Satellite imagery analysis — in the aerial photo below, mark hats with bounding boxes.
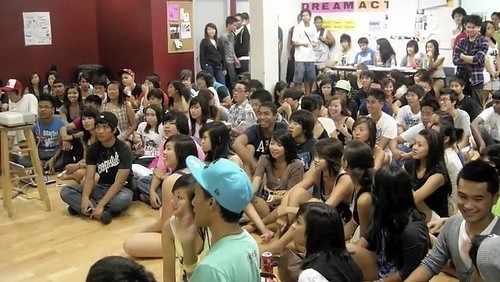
[186,155,254,213]
[476,233,500,282]
[333,80,351,92]
[119,69,134,76]
[96,111,120,136]
[1,79,22,92]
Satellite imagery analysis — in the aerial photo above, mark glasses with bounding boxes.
[232,89,247,93]
[38,105,53,109]
[405,94,416,99]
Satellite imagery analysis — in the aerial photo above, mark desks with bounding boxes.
[325,66,420,79]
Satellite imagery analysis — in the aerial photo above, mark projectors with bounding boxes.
[0,110,35,127]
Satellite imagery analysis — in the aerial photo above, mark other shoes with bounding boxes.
[140,193,150,203]
[69,206,77,214]
[101,210,112,225]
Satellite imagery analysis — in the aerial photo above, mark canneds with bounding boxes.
[261,252,273,274]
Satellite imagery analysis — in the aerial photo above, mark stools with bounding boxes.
[0,124,53,217]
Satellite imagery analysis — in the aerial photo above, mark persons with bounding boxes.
[0,63,500,282]
[373,38,397,67]
[401,41,424,67]
[422,39,446,99]
[452,7,500,107]
[354,37,378,65]
[336,33,356,65]
[285,9,335,95]
[199,13,249,86]
